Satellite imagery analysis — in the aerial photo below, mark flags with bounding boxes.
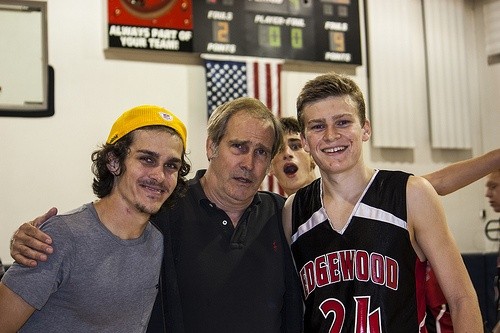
[205,59,285,198]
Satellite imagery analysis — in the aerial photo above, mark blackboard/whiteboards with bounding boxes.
[0,0,48,111]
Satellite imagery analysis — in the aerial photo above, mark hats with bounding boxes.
[106,104,187,152]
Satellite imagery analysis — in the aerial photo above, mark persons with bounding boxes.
[265,116,500,333]
[10,96,306,333]
[1,106,191,333]
[281,74,484,333]
